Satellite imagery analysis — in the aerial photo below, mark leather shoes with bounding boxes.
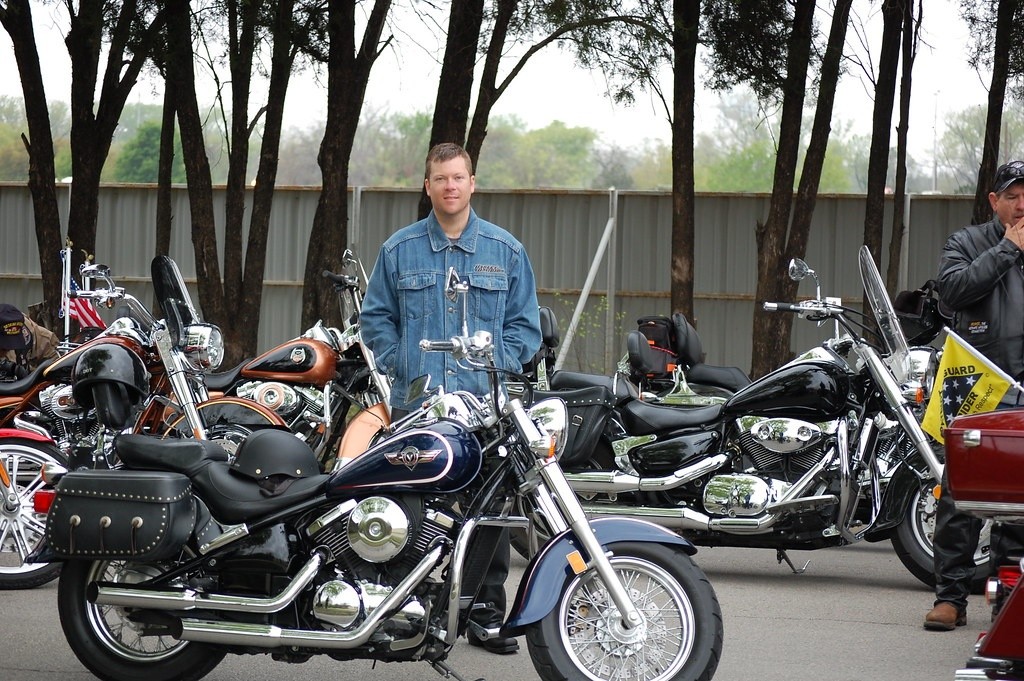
[466,622,520,653]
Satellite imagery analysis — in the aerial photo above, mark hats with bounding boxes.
[992,160,1024,193]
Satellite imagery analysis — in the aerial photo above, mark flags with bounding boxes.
[920,329,1012,446]
[58,272,106,329]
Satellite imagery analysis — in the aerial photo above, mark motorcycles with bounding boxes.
[0,235,1024,681]
[27,265,724,680]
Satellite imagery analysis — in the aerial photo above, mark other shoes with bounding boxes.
[923,601,967,629]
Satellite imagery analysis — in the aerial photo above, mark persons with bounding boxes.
[0,302,61,385]
[923,160,1024,630]
[357,141,543,653]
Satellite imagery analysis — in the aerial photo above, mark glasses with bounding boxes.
[992,165,1024,190]
